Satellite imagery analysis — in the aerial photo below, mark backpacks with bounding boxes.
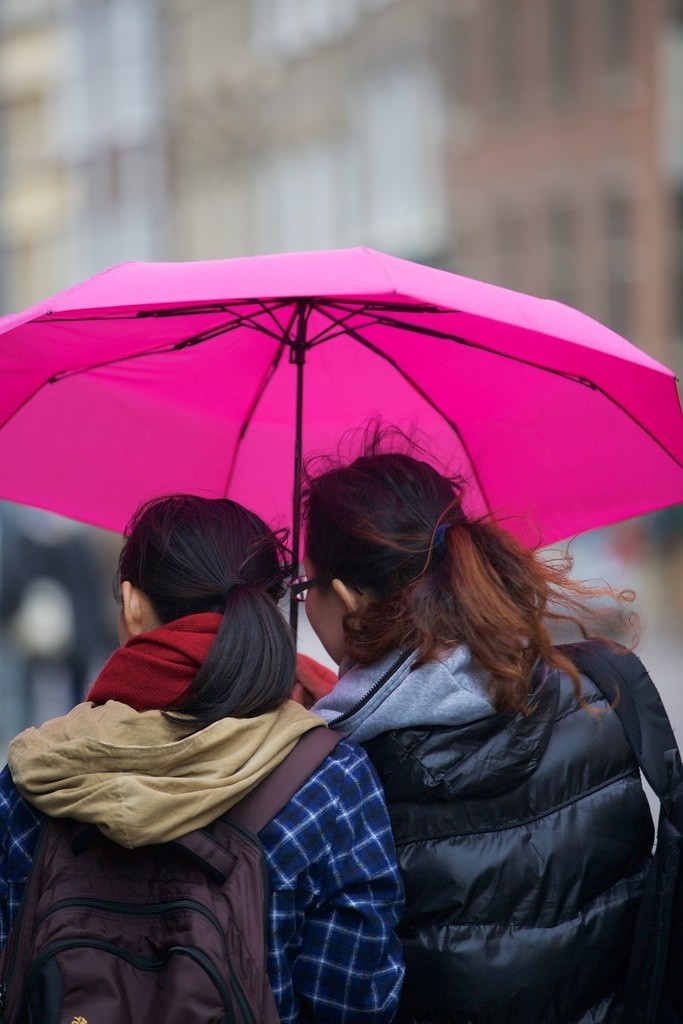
[5,727,343,1024]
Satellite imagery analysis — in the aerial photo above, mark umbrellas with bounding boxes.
[0,241,683,686]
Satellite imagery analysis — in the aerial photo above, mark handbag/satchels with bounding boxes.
[554,640,683,1024]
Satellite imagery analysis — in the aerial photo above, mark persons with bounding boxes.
[283,409,682,1023]
[0,492,407,1024]
[0,496,125,752]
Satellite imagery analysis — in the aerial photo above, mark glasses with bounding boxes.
[287,574,365,602]
